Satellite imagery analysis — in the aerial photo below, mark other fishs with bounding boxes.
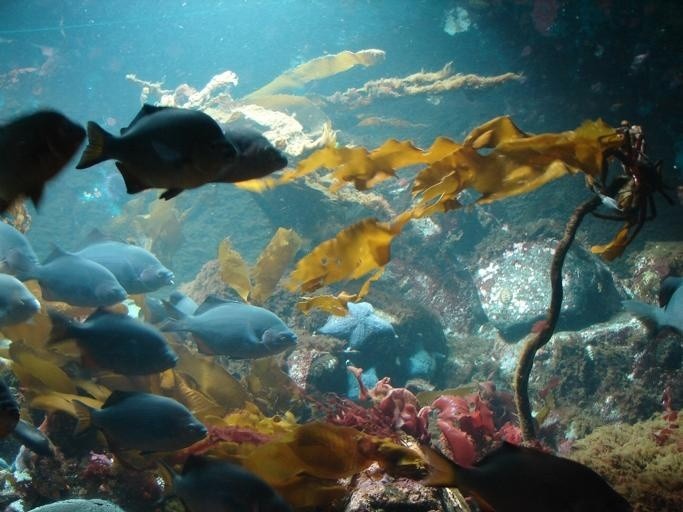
[0,102,83,217]
[78,102,293,201]
[0,226,298,508]
